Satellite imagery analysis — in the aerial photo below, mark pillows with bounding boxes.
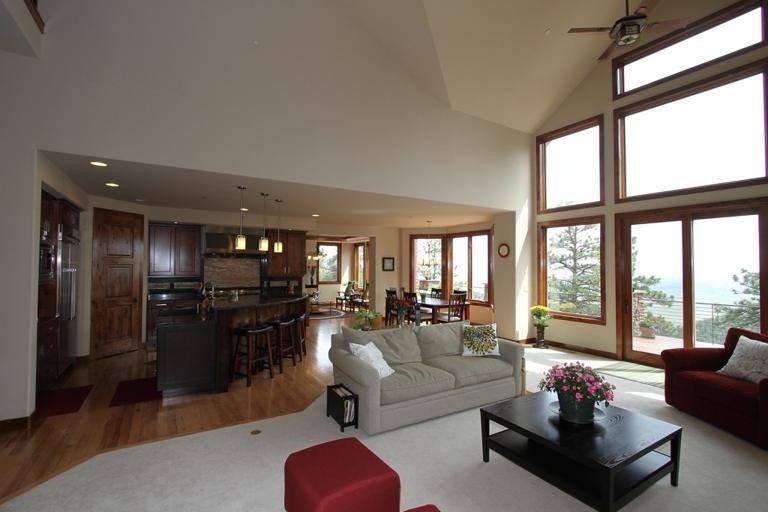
[460,322,502,357]
[348,341,396,381]
[715,334,768,385]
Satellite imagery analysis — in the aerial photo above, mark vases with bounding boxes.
[360,317,373,331]
[554,391,595,420]
[532,323,549,349]
[397,310,406,328]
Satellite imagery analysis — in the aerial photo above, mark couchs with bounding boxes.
[329,320,524,437]
[284,436,440,512]
[661,327,768,452]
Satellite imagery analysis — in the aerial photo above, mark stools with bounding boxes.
[258,313,297,375]
[353,299,362,312]
[229,321,275,387]
[336,296,345,310]
[286,311,307,362]
[363,299,370,312]
[354,294,361,299]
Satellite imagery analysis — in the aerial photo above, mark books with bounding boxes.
[333,386,355,424]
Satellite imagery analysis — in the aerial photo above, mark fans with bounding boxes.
[566,2,691,61]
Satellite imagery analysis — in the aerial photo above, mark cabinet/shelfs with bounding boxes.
[149,223,201,277]
[326,382,359,432]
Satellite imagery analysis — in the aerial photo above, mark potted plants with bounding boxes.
[311,290,319,305]
[353,305,380,320]
[222,290,239,302]
[422,270,433,281]
[639,319,658,338]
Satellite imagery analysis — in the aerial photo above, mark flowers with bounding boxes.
[558,302,577,312]
[396,298,412,312]
[528,304,552,325]
[537,361,616,408]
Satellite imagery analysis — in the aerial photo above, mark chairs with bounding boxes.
[385,289,399,327]
[441,289,467,317]
[437,292,467,323]
[390,287,404,300]
[403,291,432,325]
[420,288,444,324]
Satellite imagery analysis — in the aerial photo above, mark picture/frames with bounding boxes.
[382,256,395,272]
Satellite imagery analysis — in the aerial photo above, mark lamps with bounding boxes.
[234,186,247,251]
[259,191,270,252]
[609,14,648,47]
[274,197,284,254]
[307,238,327,261]
[418,222,440,265]
